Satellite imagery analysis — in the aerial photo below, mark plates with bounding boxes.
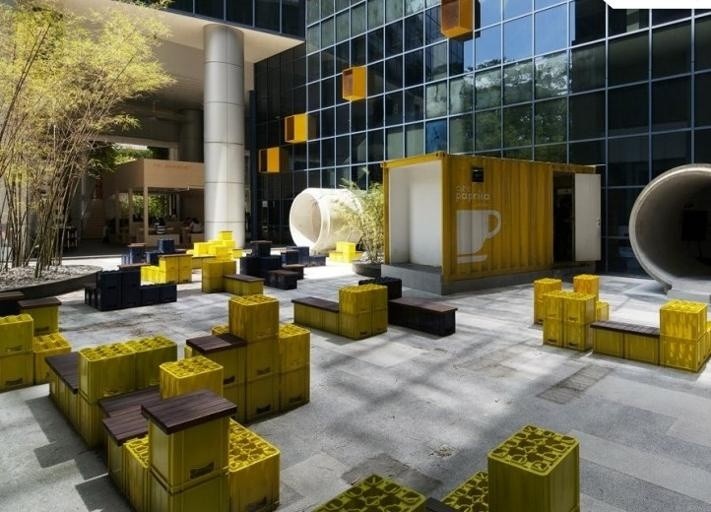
[457,255,490,264]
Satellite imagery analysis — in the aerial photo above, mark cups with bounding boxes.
[457,209,502,254]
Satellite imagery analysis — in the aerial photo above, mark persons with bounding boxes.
[149,208,204,234]
